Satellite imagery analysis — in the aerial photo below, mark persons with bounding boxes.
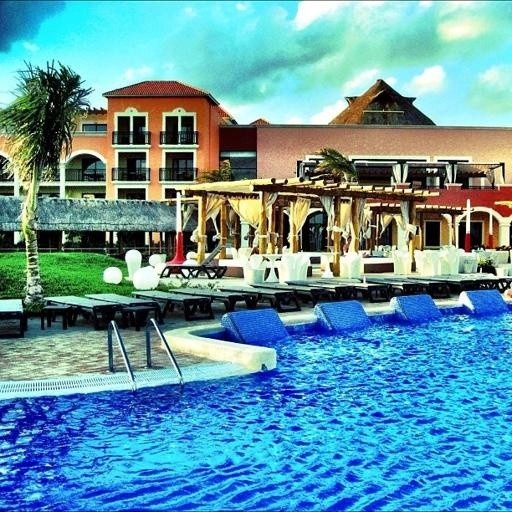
[342,236,352,256]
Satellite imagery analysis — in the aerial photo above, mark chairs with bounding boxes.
[160,245,228,279]
